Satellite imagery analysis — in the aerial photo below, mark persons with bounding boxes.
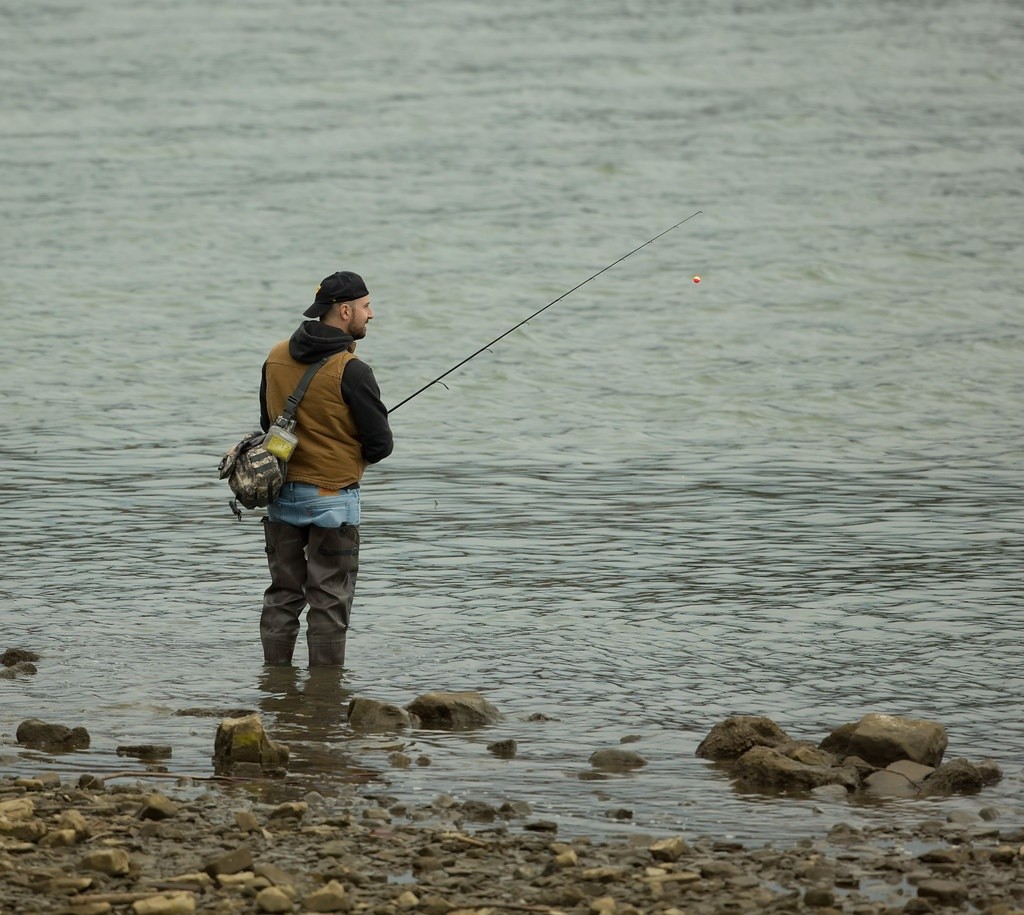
[260,271,395,664]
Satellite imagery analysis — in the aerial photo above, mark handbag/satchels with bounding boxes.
[219,430,288,522]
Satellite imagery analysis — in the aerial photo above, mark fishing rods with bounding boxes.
[387,209,704,414]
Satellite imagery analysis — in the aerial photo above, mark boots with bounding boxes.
[261,516,307,666]
[307,522,360,668]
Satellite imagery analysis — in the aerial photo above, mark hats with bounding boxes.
[303,271,369,318]
